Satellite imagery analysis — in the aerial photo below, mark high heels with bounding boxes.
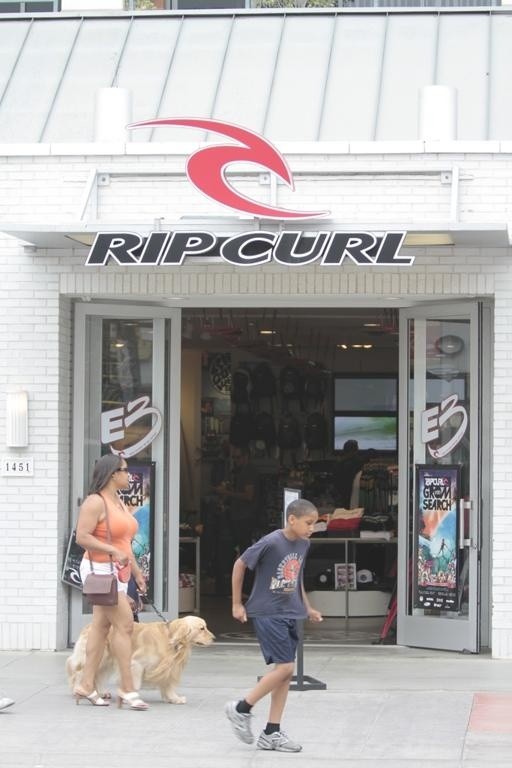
[117,688,149,711]
[74,687,110,706]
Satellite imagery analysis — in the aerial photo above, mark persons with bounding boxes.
[225,499,323,753]
[72,454,150,710]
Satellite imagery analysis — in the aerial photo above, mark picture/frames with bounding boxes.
[416,464,460,611]
[95,459,156,604]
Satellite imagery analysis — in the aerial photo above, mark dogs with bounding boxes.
[64,616,216,705]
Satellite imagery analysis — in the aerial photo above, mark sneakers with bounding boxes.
[226,700,254,744]
[257,730,302,752]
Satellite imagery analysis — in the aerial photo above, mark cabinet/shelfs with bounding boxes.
[301,531,398,618]
[180,537,200,615]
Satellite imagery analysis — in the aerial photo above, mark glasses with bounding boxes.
[118,468,129,474]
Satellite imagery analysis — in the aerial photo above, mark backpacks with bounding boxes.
[230,365,327,466]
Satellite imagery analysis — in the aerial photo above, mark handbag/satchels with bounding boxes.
[83,573,118,606]
